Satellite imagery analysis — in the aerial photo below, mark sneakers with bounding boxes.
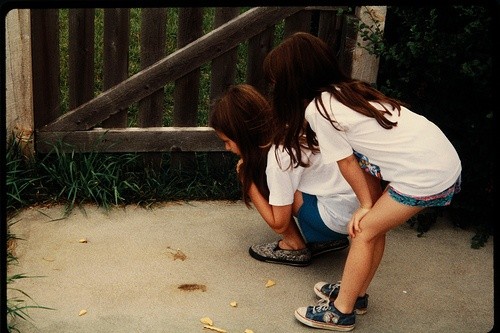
[313,281,370,315]
[294,300,357,331]
[248,238,312,268]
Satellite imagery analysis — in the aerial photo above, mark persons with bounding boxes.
[209,83,365,268]
[265,32,461,332]
